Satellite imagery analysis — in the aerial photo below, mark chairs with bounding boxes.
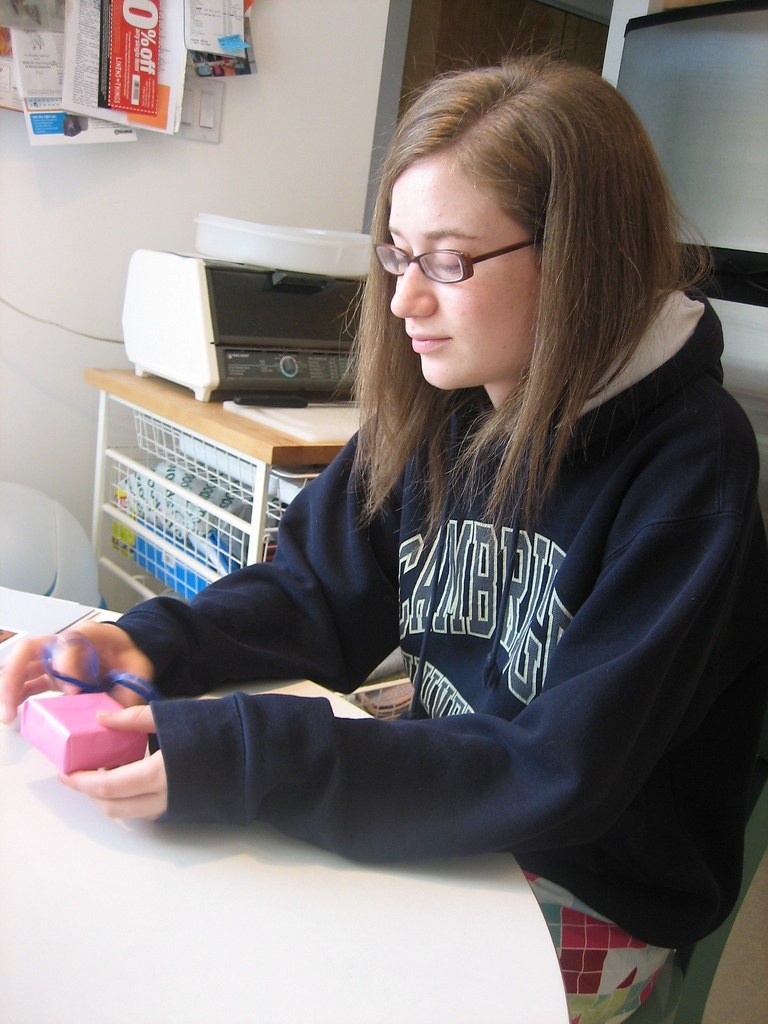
[635,425,768,1021]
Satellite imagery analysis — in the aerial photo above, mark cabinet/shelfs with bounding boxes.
[83,367,385,602]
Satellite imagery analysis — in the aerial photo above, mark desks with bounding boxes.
[4,586,568,1024]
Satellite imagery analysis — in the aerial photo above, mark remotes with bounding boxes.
[232,393,308,407]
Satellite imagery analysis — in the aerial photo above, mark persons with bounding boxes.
[1,56,767,1024]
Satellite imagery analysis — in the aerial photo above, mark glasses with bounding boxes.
[374,234,541,283]
